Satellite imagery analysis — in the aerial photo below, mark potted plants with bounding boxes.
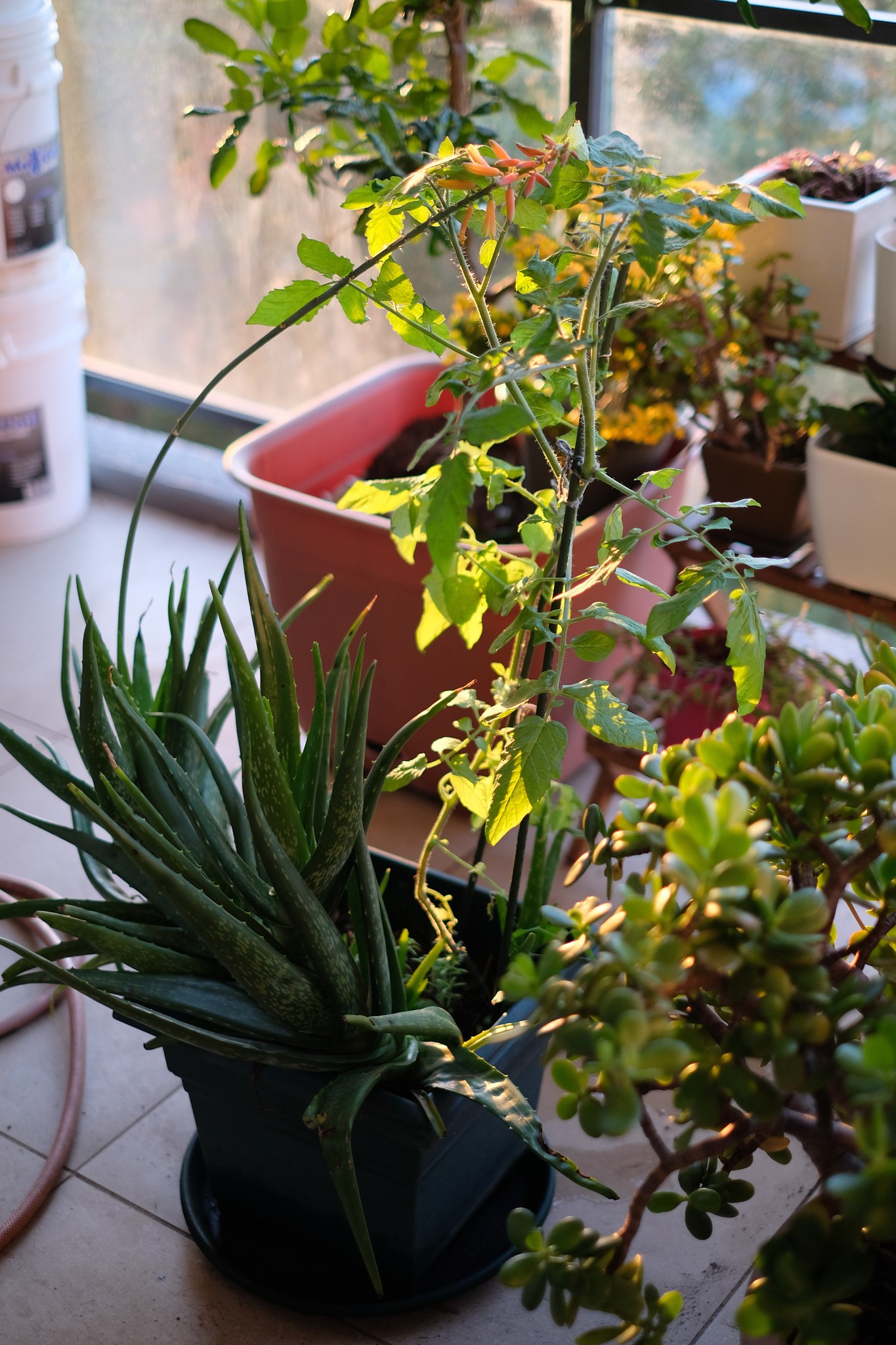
[0,0,896,1345]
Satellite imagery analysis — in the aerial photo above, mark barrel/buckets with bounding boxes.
[0,1,93,548]
